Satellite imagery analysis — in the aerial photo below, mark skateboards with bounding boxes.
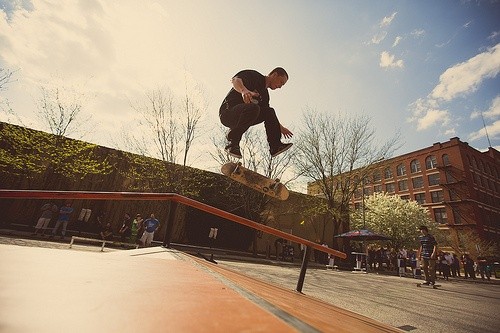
[221,162,289,201]
[416,282,443,290]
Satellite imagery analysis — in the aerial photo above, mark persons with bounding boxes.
[30,198,133,243]
[207,224,219,249]
[128,214,144,244]
[137,213,160,248]
[418,225,437,285]
[270,235,500,283]
[218,67,294,159]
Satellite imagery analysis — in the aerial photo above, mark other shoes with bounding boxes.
[224,143,242,158]
[269,142,293,157]
